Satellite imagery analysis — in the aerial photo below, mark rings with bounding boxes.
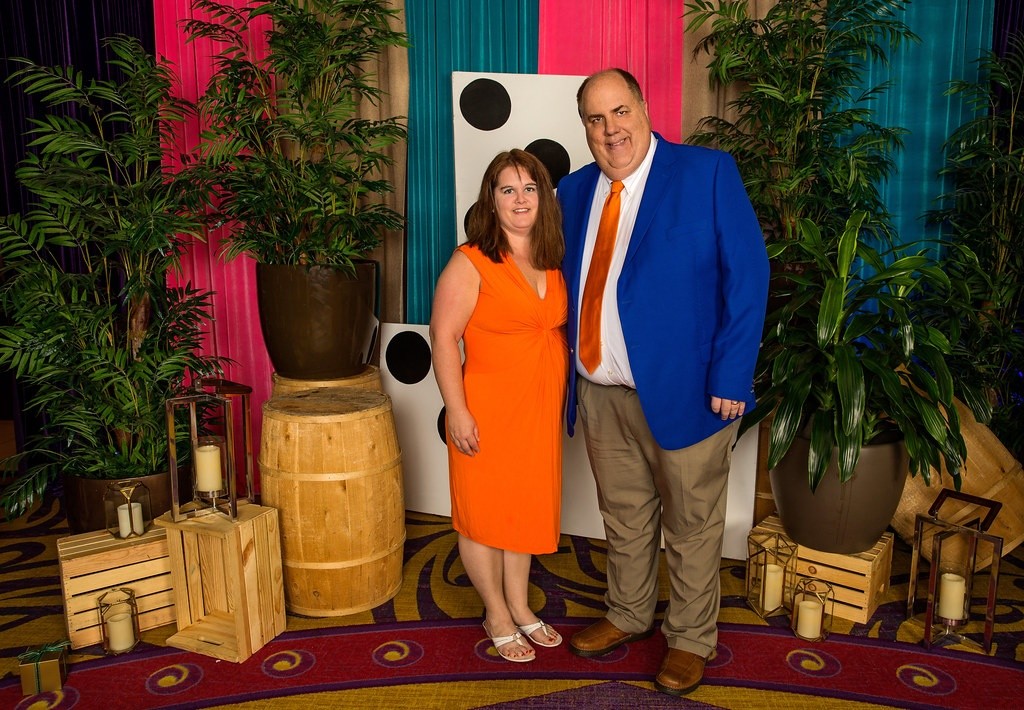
[730,399,739,406]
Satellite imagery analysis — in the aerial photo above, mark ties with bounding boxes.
[579,180,624,374]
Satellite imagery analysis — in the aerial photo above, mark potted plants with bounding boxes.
[165,0,414,383]
[0,35,230,537]
[661,0,1024,554]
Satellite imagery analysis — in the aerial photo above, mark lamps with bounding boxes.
[103,480,154,540]
[792,576,835,644]
[164,377,255,521]
[746,531,797,619]
[96,587,142,657]
[906,487,1004,655]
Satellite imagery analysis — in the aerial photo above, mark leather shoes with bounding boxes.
[570,616,656,657]
[655,647,707,695]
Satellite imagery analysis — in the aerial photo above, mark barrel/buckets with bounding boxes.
[881,362,1024,576]
[255,386,406,617]
[270,363,384,398]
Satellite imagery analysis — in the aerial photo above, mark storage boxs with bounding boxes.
[18,639,71,696]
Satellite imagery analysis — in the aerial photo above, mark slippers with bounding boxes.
[483,620,535,662]
[516,619,562,647]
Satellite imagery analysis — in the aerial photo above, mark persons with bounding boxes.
[430,150,570,663]
[556,69,769,696]
